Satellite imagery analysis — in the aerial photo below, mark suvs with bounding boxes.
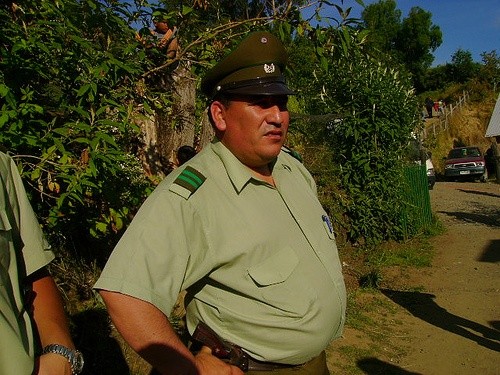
[444,147,488,182]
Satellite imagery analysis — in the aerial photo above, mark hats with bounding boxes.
[200,31,297,99]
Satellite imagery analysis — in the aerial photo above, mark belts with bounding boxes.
[240,355,305,371]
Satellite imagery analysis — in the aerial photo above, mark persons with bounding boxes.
[420,96,445,120]
[0,152,85,375]
[151,9,177,57]
[95,30,346,375]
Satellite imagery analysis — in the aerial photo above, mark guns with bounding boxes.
[188,320,249,373]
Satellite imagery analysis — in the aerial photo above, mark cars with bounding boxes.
[416,156,436,191]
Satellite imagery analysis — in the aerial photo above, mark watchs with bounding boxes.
[41,344,84,375]
